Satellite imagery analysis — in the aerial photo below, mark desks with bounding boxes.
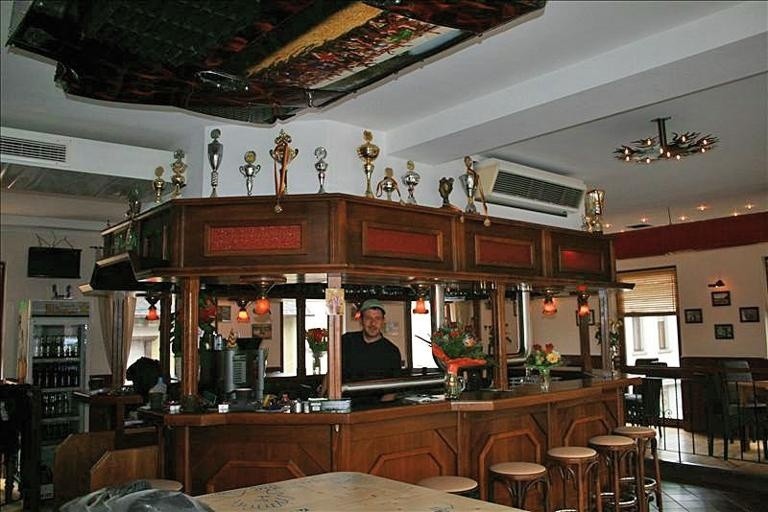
[189,470,522,512]
[72,388,143,432]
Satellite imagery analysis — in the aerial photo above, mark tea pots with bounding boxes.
[443,373,466,400]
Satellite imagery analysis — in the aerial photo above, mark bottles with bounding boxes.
[167,388,311,413]
[31,335,78,438]
[199,333,224,351]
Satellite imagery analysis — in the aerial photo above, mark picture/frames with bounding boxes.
[573,309,595,327]
[737,306,759,324]
[712,324,733,341]
[709,289,731,308]
[683,308,702,325]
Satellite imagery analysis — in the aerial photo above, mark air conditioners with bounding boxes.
[471,155,589,216]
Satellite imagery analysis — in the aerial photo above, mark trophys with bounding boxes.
[585,190,604,233]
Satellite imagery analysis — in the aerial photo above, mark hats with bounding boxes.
[360,299,388,317]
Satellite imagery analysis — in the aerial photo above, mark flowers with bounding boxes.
[424,313,499,369]
[169,280,222,337]
[527,340,565,370]
[304,324,330,353]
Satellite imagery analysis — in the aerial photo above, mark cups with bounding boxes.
[520,368,538,384]
[149,392,163,409]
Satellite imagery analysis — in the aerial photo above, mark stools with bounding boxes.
[546,446,603,512]
[610,426,664,512]
[415,476,479,498]
[486,462,552,512]
[142,477,183,496]
[586,435,644,511]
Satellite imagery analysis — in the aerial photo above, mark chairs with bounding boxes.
[636,354,766,465]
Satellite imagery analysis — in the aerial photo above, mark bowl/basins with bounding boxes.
[235,338,262,348]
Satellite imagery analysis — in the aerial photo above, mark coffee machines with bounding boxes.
[199,349,267,400]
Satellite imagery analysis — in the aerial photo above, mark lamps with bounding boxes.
[351,296,366,321]
[535,283,565,316]
[573,288,593,318]
[612,114,719,165]
[229,289,253,324]
[143,292,163,322]
[241,273,287,320]
[411,282,430,317]
[707,279,726,288]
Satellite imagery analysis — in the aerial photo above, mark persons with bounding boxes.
[316,296,400,404]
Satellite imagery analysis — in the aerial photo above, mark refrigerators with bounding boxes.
[17,298,92,501]
